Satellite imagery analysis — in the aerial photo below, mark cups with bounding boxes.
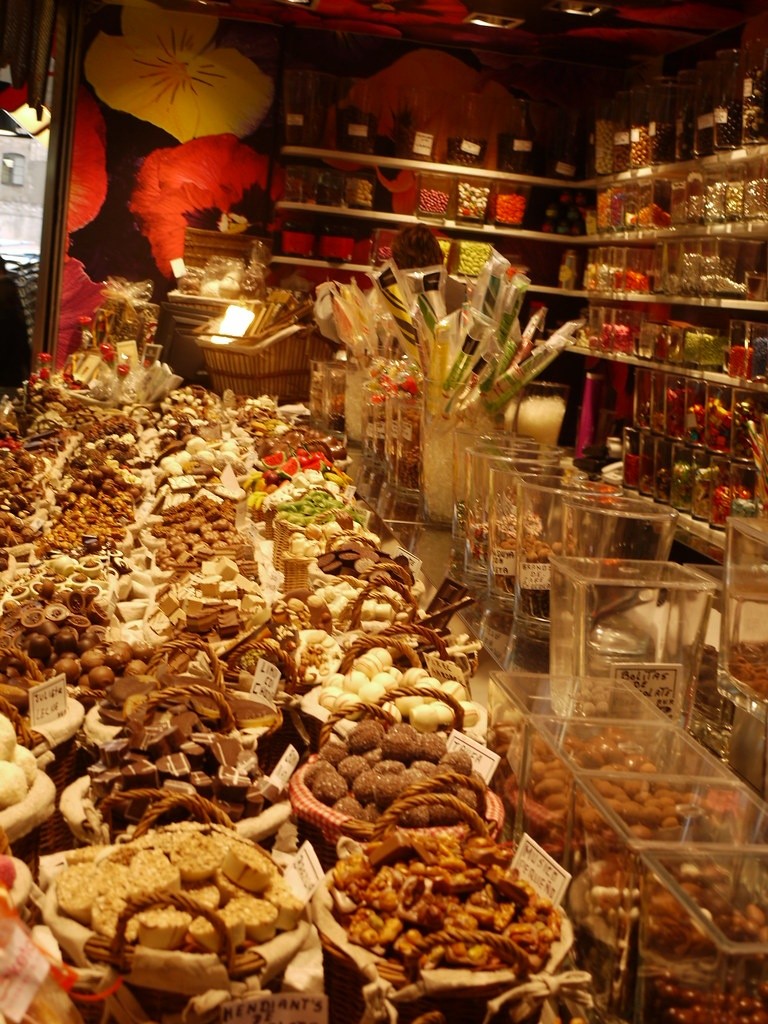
[308,358,678,641]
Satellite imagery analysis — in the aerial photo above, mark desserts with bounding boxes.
[0,372,562,972]
[486,717,767,1024]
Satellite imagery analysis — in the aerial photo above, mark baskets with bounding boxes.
[164,226,339,402]
[0,384,562,990]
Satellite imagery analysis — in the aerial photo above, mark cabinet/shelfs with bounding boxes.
[269,146,768,567]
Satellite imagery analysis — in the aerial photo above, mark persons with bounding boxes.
[392,223,465,316]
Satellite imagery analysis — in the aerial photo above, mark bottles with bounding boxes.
[269,60,768,534]
